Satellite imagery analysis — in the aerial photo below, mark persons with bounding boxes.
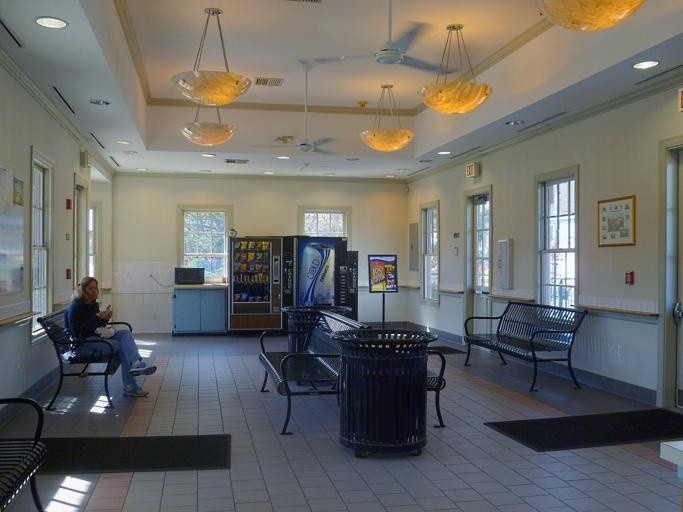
[67,276,157,397]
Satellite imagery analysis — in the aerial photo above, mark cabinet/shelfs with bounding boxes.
[229,236,283,330]
[170,283,230,335]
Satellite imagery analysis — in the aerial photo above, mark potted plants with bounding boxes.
[229,229,237,238]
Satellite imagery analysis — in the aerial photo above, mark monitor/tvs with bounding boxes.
[175,268,204,285]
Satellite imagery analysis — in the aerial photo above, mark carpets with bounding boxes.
[483,408,683,452]
[1,434,232,471]
[428,345,466,355]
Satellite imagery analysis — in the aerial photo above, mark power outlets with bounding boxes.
[148,272,154,279]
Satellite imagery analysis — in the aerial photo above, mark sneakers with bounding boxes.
[123,383,149,397]
[129,361,157,376]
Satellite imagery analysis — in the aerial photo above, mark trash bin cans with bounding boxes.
[330,327,439,457]
[284,304,352,354]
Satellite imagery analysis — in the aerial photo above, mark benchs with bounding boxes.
[0,398,48,512]
[427,350,446,427]
[259,309,370,435]
[38,311,132,409]
[465,301,587,393]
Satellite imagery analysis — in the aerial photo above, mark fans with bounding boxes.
[250,67,339,163]
[315,0,457,75]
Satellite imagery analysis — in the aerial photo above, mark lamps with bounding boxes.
[536,0,647,32]
[180,103,234,147]
[172,7,251,104]
[422,23,493,115]
[359,85,415,152]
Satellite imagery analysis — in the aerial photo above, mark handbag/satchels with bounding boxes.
[79,346,101,362]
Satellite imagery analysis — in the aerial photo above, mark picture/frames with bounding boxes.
[368,254,398,293]
[597,194,636,246]
